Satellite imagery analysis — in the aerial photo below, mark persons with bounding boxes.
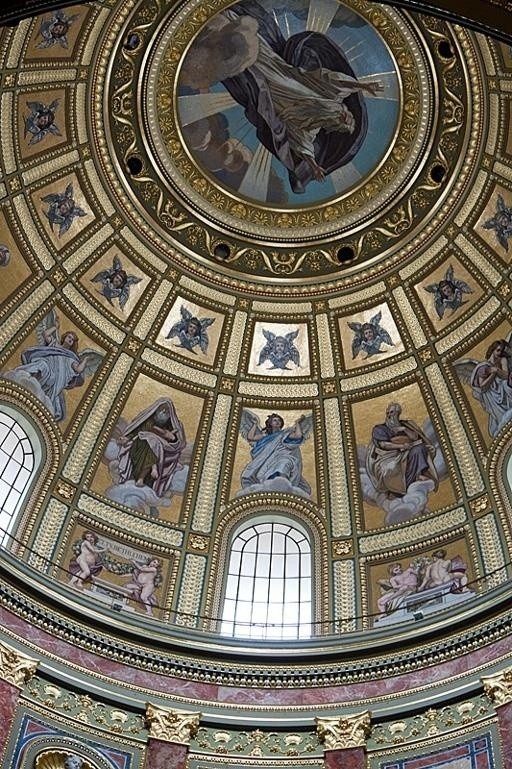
[13,21,512,622]
